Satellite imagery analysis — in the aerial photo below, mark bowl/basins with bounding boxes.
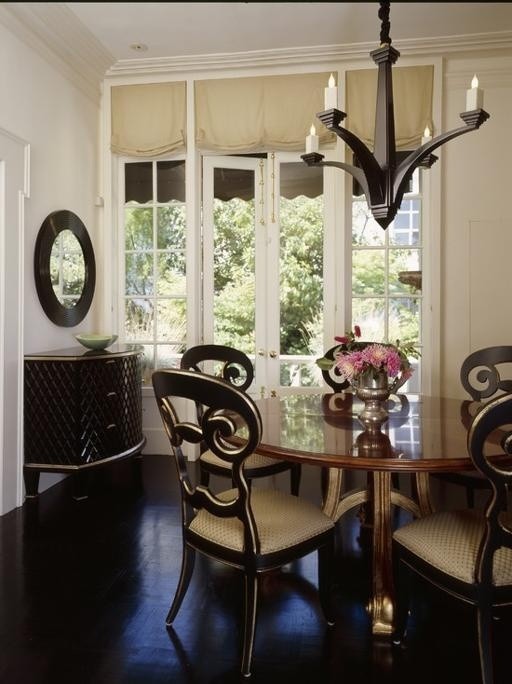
[75,334,119,350]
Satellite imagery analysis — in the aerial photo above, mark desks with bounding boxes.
[222,391,511,651]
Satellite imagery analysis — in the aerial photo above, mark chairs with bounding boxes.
[317,341,414,396]
[151,366,344,678]
[390,392,512,684]
[178,344,304,496]
[459,342,512,397]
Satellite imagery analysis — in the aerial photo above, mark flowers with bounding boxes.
[332,326,423,381]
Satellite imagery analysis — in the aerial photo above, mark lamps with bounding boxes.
[301,2,490,232]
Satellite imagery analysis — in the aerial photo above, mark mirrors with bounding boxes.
[34,209,97,330]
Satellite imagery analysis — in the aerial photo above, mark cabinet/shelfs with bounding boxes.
[24,342,147,499]
